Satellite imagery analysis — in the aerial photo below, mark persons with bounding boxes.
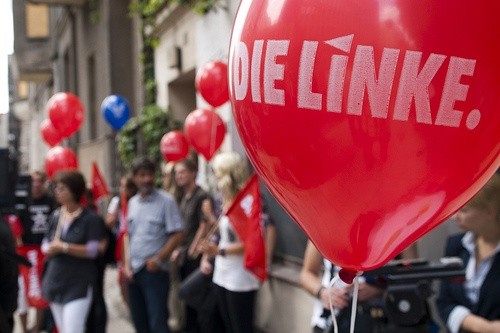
[7,169,117,333]
[434,168,500,333]
[299,237,385,333]
[106,174,138,306]
[173,158,216,333]
[119,158,188,333]
[199,151,276,333]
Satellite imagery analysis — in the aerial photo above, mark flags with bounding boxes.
[91,166,109,202]
[223,175,267,283]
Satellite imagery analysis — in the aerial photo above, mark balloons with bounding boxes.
[198,62,229,108]
[160,131,189,162]
[40,119,62,148]
[184,109,227,161]
[44,145,78,180]
[100,94,131,132]
[227,0,500,284]
[47,93,83,138]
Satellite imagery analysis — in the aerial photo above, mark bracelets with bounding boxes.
[315,285,326,298]
[220,248,226,256]
[62,243,69,253]
